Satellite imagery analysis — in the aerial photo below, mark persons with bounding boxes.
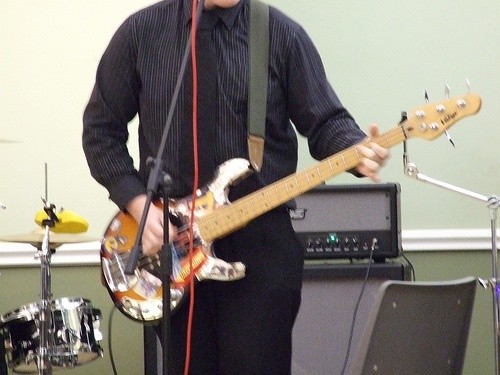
[82,0,390,375]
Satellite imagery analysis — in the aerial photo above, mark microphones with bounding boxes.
[168,214,183,229]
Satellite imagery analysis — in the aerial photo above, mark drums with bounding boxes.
[7,298,93,319]
[4,308,104,373]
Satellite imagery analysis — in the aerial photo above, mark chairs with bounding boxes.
[292,275,477,375]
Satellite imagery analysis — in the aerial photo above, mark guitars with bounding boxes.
[100,78,482,323]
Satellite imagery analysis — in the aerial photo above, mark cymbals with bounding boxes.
[1,229,100,248]
